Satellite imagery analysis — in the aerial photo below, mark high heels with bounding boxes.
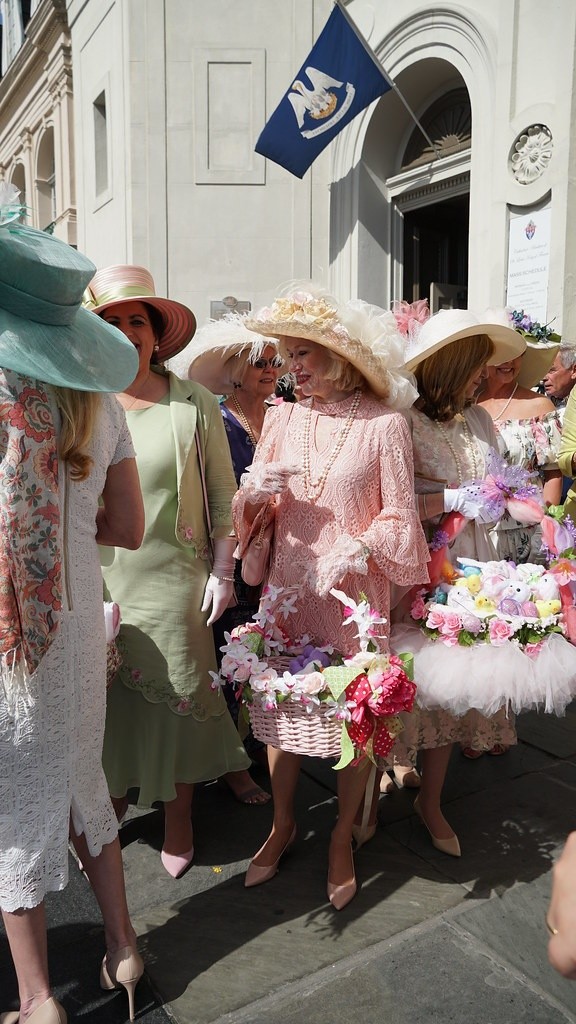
[0,995,67,1024]
[328,833,357,911]
[98,945,143,1023]
[413,793,462,857]
[352,805,376,844]
[245,819,298,888]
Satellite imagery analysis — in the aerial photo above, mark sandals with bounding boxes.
[227,772,270,807]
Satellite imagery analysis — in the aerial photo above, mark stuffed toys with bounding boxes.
[441,556,562,616]
[278,645,329,676]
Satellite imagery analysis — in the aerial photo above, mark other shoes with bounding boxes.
[461,736,507,756]
[380,766,424,794]
[159,826,193,880]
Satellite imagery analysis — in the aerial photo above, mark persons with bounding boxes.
[544,834,576,979]
[79,265,576,912]
[0,212,143,1024]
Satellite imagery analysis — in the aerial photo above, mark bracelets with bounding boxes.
[209,573,235,582]
[423,494,429,520]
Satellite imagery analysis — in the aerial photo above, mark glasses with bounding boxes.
[250,357,283,370]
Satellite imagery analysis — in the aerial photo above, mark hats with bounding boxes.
[399,310,526,374]
[502,308,560,392]
[185,310,286,396]
[77,266,197,366]
[0,200,139,392]
[242,284,419,408]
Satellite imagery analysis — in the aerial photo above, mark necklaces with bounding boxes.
[428,405,477,487]
[302,390,363,502]
[473,381,520,420]
[229,391,271,450]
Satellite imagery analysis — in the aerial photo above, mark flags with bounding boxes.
[255,0,395,179]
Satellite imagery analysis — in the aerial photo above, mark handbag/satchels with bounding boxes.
[240,505,275,587]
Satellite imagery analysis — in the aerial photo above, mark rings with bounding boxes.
[546,916,557,934]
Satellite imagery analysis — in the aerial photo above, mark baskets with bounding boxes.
[235,582,372,759]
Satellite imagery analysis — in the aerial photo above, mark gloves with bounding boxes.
[443,486,506,524]
[199,538,238,626]
